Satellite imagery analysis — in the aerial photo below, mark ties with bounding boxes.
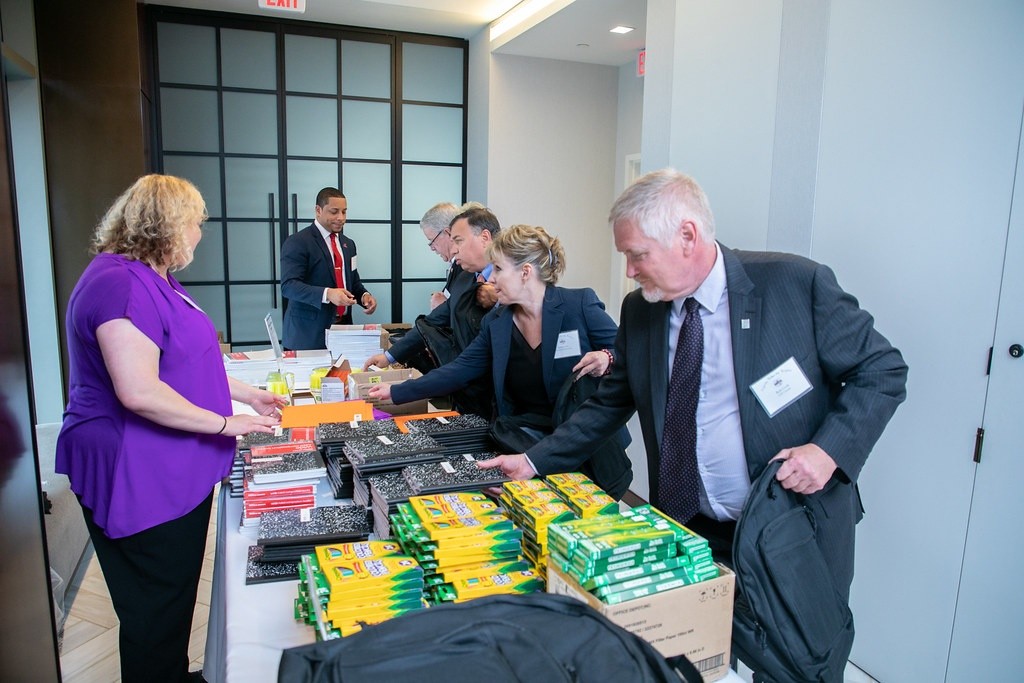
[330,233,345,317]
[656,298,704,525]
[477,274,486,284]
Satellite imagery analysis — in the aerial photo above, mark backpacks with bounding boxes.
[729,460,854,683]
[276,593,706,683]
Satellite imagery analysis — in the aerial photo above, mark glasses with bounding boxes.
[428,229,444,248]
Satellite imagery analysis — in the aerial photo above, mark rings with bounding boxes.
[270,414,273,417]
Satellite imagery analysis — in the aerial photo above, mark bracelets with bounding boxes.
[217,416,227,434]
[602,349,614,375]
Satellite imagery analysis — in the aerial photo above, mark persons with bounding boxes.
[54,173,290,683]
[363,202,501,374]
[368,227,634,501]
[280,187,377,351]
[476,167,909,673]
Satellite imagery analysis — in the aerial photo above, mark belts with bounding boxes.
[335,316,348,323]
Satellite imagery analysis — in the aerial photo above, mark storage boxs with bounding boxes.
[347,368,429,416]
[546,556,736,683]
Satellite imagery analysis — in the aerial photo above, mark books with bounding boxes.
[222,323,512,586]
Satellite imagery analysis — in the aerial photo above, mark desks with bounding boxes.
[200,392,751,683]
